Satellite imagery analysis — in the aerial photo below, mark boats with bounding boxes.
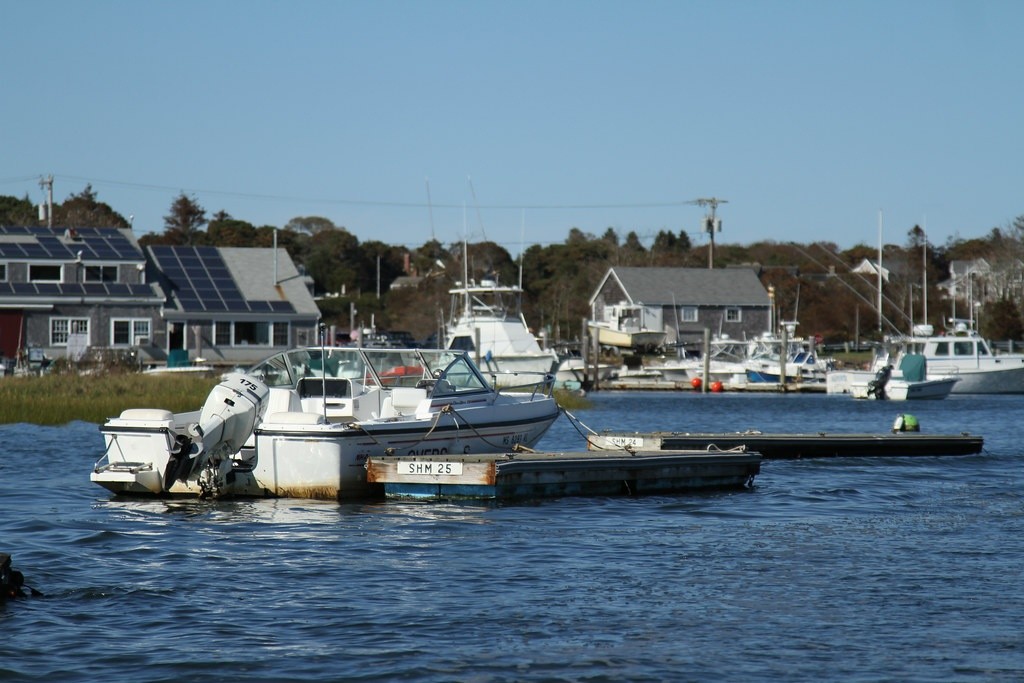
[423,224,558,385]
[644,279,831,392]
[847,354,964,402]
[557,358,612,389]
[588,299,667,351]
[90,316,560,501]
[137,367,215,377]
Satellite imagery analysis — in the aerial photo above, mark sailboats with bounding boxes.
[871,207,1024,393]
